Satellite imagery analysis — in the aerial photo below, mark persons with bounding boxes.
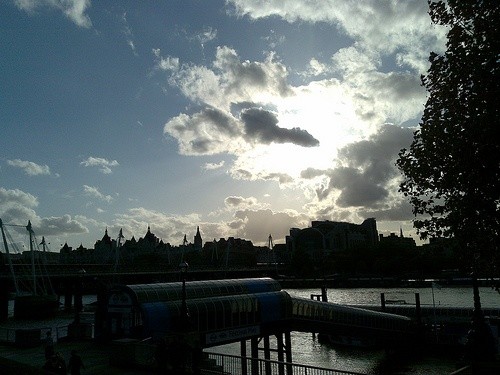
[41,330,86,374]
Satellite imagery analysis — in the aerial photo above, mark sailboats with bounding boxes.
[0,218,61,323]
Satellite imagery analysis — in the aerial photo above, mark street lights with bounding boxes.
[463,241,497,362]
[74,269,87,324]
[175,262,194,333]
